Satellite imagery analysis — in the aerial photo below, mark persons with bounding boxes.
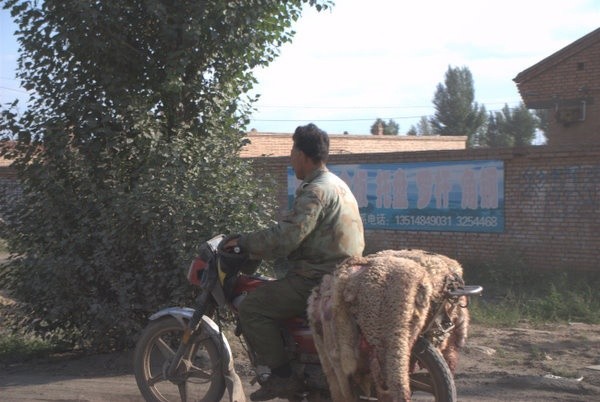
[223,123,365,401]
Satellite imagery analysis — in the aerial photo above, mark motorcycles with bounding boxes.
[132,232,484,402]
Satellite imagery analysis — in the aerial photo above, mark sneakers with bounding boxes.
[250,372,301,401]
